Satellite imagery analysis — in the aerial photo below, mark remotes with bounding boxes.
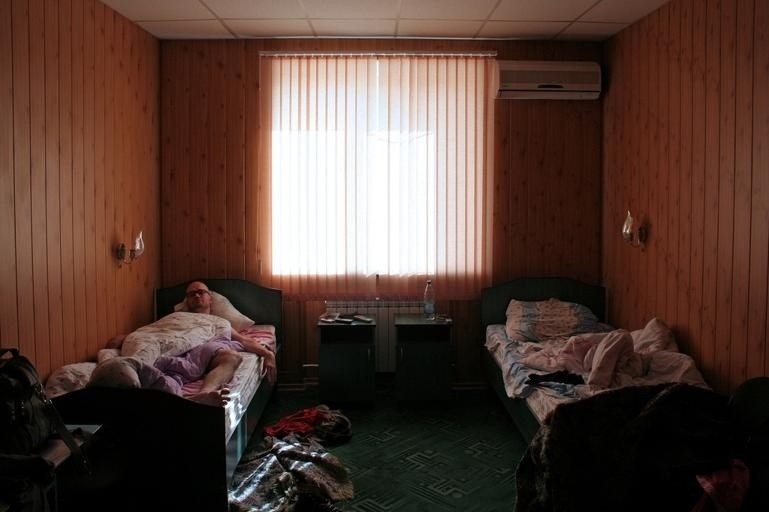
[335,317,353,323]
[353,315,373,323]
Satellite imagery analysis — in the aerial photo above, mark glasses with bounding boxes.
[186,289,210,297]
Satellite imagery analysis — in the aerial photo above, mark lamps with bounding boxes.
[619,208,646,248]
[115,229,145,268]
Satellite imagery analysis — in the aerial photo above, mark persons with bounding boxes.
[186,279,279,407]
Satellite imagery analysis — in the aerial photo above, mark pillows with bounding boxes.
[504,296,603,341]
[174,290,255,333]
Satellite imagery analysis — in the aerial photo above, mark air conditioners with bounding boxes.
[492,58,602,101]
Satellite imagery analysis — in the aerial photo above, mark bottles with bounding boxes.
[424,279,437,321]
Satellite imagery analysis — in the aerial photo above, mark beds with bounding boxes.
[478,276,769,511]
[46,279,281,510]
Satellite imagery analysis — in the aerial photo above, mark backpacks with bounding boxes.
[1,347,95,477]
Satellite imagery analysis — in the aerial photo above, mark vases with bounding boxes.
[424,278,437,314]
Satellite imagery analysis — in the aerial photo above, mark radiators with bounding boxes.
[325,301,434,374]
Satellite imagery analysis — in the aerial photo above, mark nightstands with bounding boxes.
[393,311,455,411]
[317,311,377,406]
